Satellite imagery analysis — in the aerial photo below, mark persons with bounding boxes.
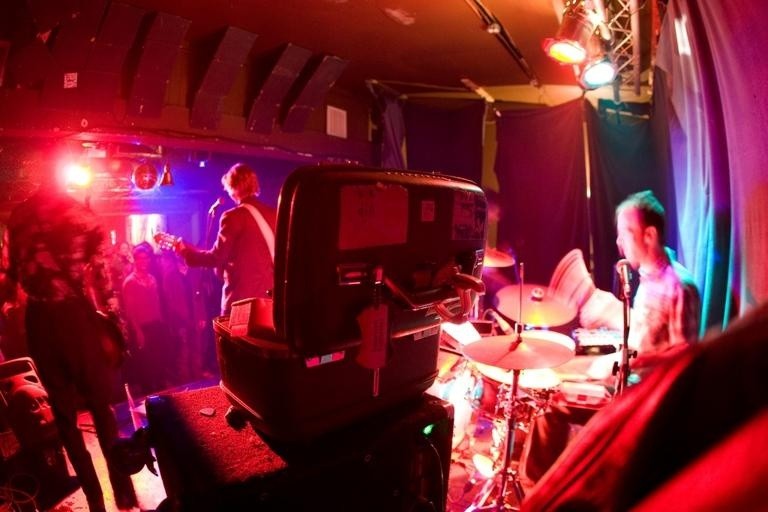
[9,246,149,512]
[526,186,703,486]
[1,232,218,389]
[173,164,279,318]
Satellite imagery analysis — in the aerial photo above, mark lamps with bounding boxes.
[542,1,605,64]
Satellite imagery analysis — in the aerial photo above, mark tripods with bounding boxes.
[463,370,526,512]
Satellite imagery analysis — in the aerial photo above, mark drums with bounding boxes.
[436,321,480,384]
[463,361,562,429]
[549,249,632,331]
[518,329,576,351]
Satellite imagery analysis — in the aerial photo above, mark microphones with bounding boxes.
[208,196,225,215]
[491,310,514,335]
[616,259,633,298]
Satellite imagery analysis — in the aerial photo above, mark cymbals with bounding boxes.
[483,248,515,268]
[463,336,574,370]
[495,283,578,327]
